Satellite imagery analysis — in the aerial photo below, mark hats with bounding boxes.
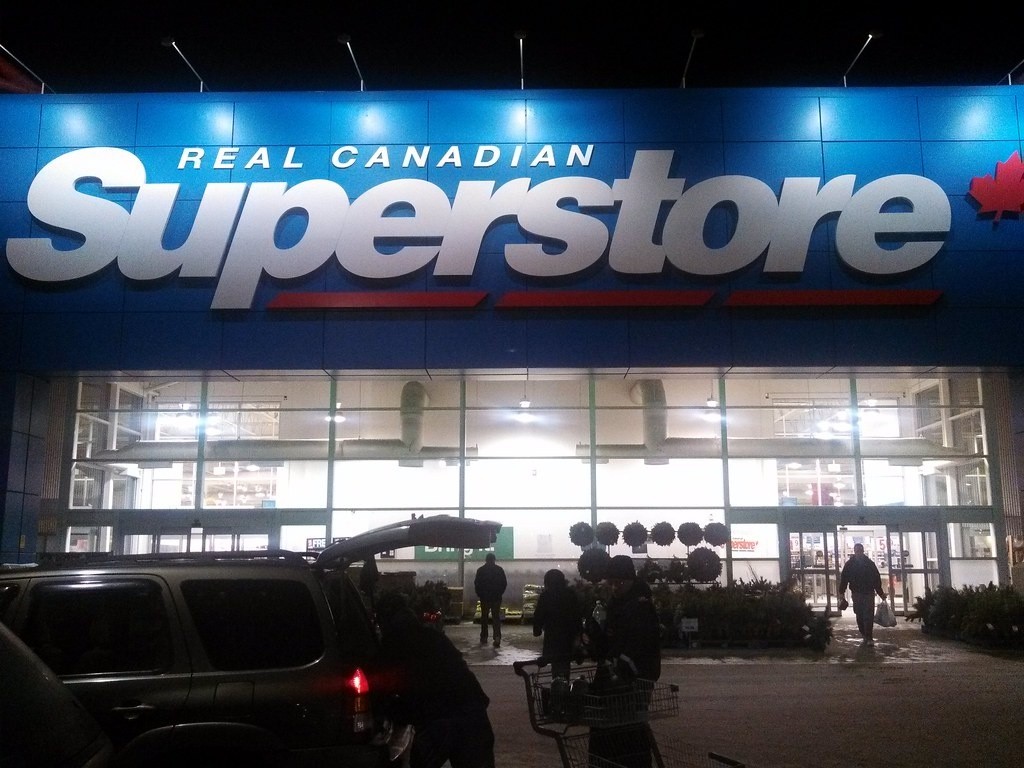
[606,556,636,580]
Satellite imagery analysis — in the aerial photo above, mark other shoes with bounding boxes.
[863,638,874,646]
[480,638,487,643]
[493,637,500,646]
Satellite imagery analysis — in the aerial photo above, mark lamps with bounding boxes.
[707,379,717,406]
[519,381,530,407]
[179,382,191,410]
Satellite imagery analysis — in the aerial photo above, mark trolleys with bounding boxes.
[513,654,746,768]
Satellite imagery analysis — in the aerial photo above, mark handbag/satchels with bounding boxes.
[874,598,897,627]
[576,640,587,665]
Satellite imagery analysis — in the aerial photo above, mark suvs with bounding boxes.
[1,514,502,768]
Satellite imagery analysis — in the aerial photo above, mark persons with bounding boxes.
[534,569,581,681]
[367,593,495,768]
[838,544,887,646]
[582,556,661,768]
[795,550,815,581]
[474,553,507,647]
